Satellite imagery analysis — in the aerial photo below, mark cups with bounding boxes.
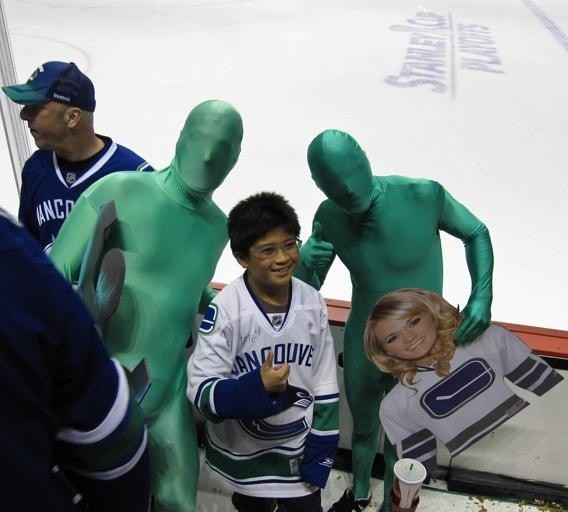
[388,457,427,512]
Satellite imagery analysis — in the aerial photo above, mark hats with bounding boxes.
[2,58,96,112]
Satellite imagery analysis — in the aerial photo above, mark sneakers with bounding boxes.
[327,486,372,512]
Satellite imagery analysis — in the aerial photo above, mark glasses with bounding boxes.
[254,237,301,260]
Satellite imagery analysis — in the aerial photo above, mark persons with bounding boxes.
[2,60,163,236]
[0,205,153,508]
[44,96,247,510]
[290,128,496,511]
[181,189,344,512]
[361,292,563,469]
